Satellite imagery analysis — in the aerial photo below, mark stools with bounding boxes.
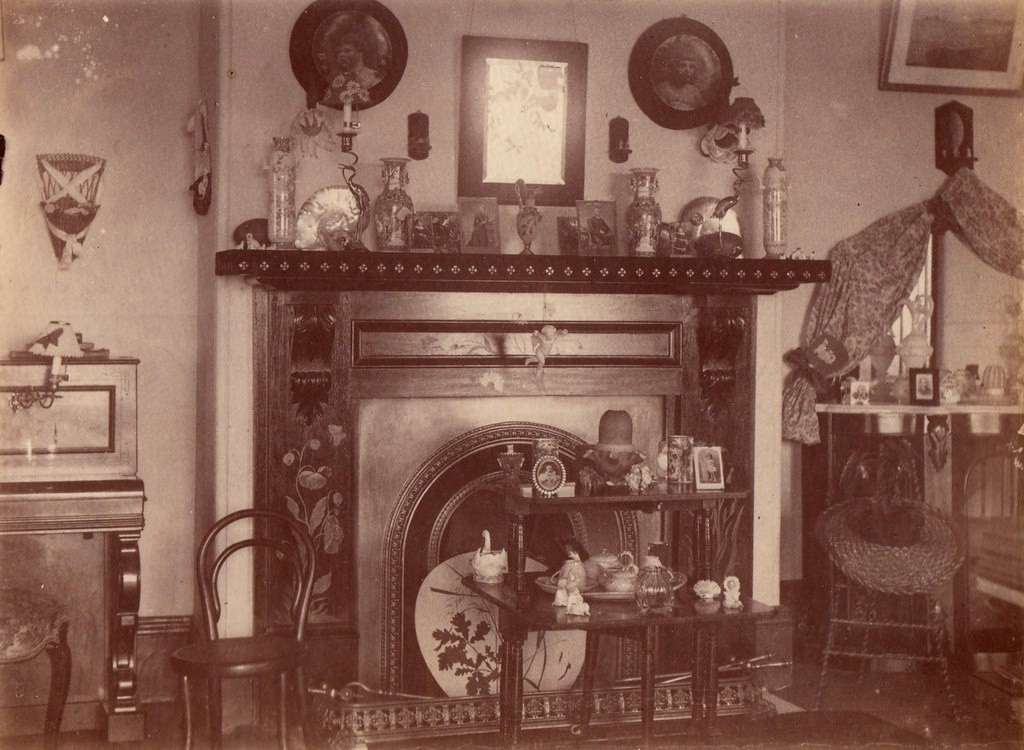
[1,590,75,750]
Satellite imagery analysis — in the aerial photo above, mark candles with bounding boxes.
[737,124,749,150]
[344,102,352,123]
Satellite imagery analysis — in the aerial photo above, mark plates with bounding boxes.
[535,575,599,595]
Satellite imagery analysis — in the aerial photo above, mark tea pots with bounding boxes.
[939,371,961,405]
[590,548,619,570]
[601,567,637,592]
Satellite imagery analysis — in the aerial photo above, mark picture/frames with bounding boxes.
[909,368,940,407]
[532,455,565,495]
[627,18,735,131]
[575,198,618,256]
[693,446,725,492]
[877,0,1024,97]
[459,32,588,208]
[289,0,409,113]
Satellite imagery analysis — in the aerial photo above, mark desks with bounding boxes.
[806,401,1023,636]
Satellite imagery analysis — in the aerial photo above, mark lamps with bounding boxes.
[10,321,80,412]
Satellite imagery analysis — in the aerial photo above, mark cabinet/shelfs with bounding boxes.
[464,490,781,750]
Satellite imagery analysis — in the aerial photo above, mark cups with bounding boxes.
[667,435,695,494]
[497,453,525,480]
[407,214,434,253]
[551,560,586,588]
[642,556,673,581]
[635,573,675,612]
[953,369,974,399]
[532,438,559,500]
[557,217,580,256]
[979,365,1005,395]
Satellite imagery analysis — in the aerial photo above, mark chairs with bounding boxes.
[170,503,315,750]
[814,440,1020,727]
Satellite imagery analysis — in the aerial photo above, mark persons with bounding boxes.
[525,325,568,380]
[703,450,717,482]
[539,464,558,486]
[918,378,931,395]
[413,219,431,248]
[588,208,615,252]
[329,34,379,90]
[467,205,489,247]
[552,579,591,616]
[659,54,702,110]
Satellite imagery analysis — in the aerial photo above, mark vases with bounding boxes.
[374,157,413,250]
[627,167,664,258]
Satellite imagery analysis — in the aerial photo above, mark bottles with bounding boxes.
[270,136,297,242]
[626,167,663,258]
[763,158,789,259]
[515,179,543,254]
[375,158,413,252]
